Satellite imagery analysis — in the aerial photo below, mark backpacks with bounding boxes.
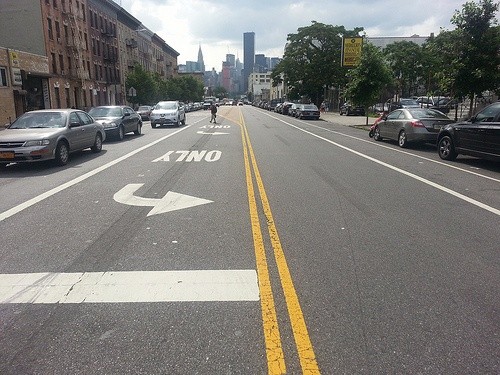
[212,106,217,113]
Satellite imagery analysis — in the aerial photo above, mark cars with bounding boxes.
[183,97,321,120]
[339,101,367,116]
[0,108,106,170]
[435,101,500,162]
[88,105,143,141]
[374,95,458,114]
[150,101,186,129]
[372,108,457,148]
[136,106,152,121]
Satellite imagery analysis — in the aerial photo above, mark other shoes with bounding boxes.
[214,122,216,123]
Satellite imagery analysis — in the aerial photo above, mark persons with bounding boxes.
[209,101,217,123]
[321,101,329,113]
